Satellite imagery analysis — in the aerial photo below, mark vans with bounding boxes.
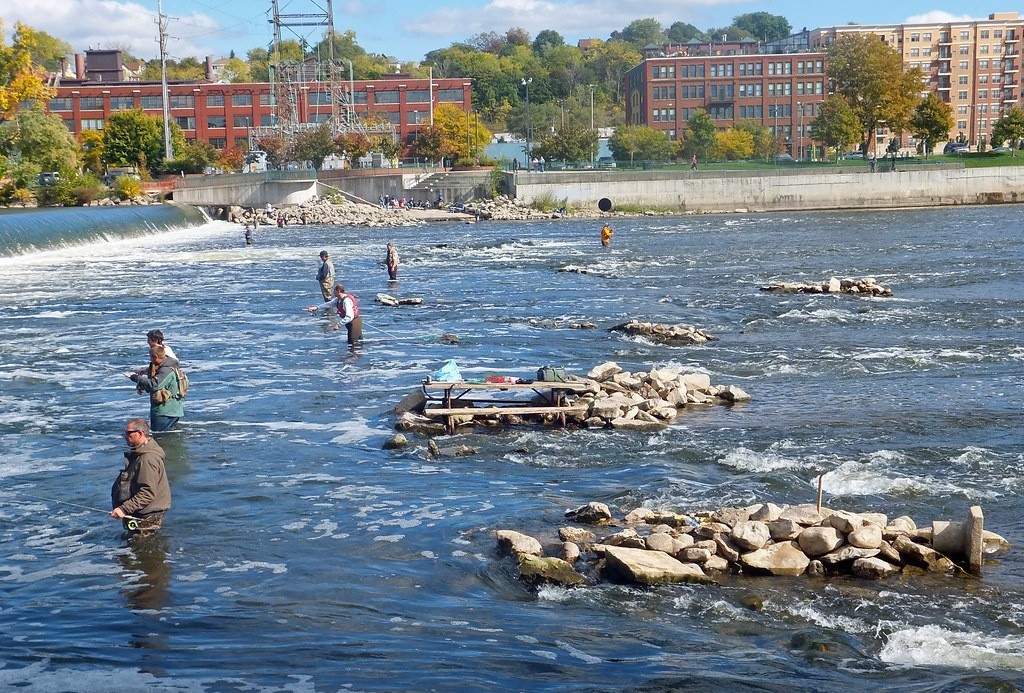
[34,172,69,185]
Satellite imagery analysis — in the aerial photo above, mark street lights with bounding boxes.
[556,100,568,170]
[586,84,597,169]
[521,77,533,172]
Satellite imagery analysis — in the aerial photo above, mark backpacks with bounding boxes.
[164,367,189,398]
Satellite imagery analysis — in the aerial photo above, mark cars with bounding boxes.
[943,143,967,154]
[844,151,863,160]
[598,157,617,168]
[989,146,1016,154]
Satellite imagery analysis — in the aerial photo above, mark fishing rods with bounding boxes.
[11,490,158,530]
[361,321,397,338]
[376,261,386,270]
[254,308,309,311]
[8,330,138,378]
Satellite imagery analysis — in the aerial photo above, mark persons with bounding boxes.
[245,227,253,246]
[870,157,877,173]
[315,250,335,302]
[278,211,289,226]
[533,157,539,172]
[691,155,699,171]
[131,328,190,434]
[265,202,272,218]
[514,158,519,173]
[378,193,446,211]
[539,156,546,172]
[307,285,363,343]
[892,154,896,171]
[109,421,173,537]
[300,210,308,225]
[601,222,613,247]
[386,243,399,278]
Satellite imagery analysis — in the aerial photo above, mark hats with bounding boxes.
[386,243,393,247]
[319,251,329,257]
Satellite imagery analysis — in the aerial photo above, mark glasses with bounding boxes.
[125,430,144,434]
